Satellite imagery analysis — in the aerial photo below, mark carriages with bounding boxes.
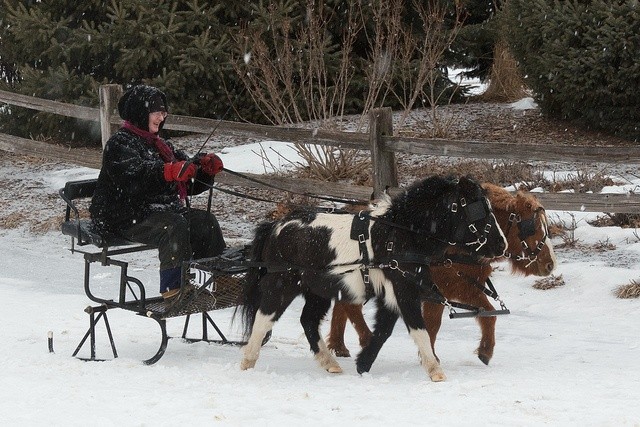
[49,174,557,381]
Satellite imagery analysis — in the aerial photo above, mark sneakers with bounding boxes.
[162,283,195,305]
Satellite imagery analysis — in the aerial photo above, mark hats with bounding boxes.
[149,94,166,112]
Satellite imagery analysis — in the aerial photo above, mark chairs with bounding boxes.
[58,174,214,308]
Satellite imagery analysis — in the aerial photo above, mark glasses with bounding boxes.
[153,112,167,118]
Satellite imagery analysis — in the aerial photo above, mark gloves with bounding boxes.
[164,160,197,182]
[201,153,223,176]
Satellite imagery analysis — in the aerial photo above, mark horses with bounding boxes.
[325,182,556,365]
[232,173,508,382]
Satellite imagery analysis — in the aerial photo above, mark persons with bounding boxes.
[88,84,223,302]
